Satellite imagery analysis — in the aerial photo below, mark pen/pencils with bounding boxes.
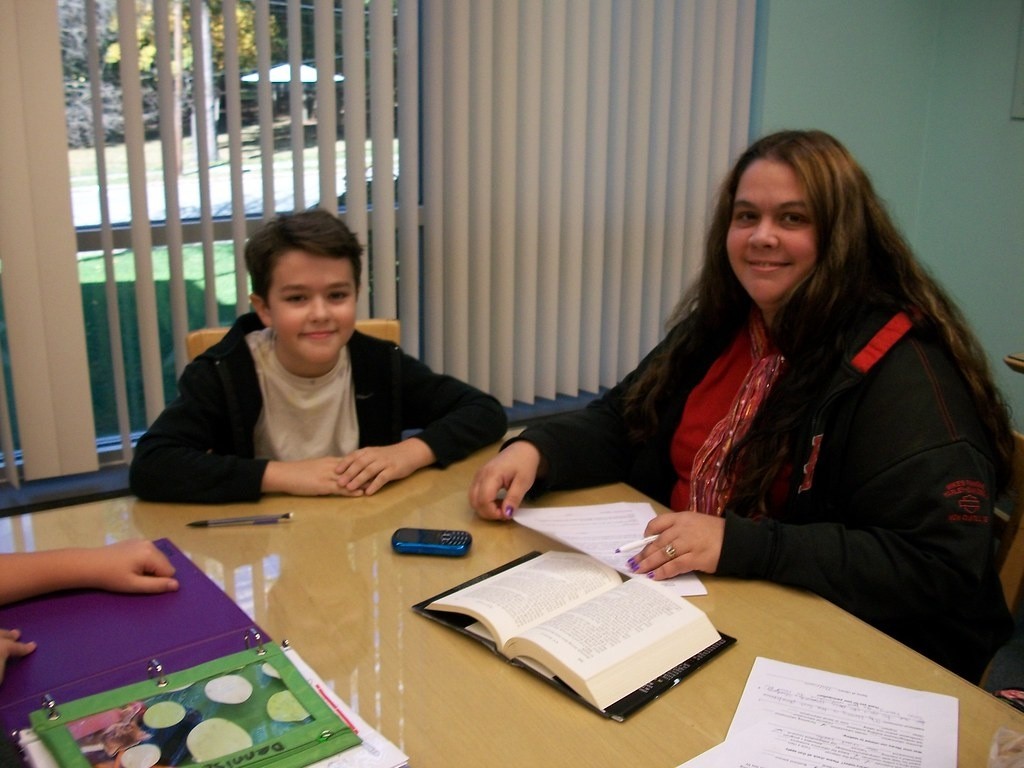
[185,513,292,528]
[614,534,659,553]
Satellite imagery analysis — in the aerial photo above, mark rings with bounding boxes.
[666,545,676,558]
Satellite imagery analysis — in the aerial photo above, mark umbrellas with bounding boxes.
[241,63,345,83]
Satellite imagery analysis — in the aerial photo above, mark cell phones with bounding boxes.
[994,687,1024,712]
[391,528,472,556]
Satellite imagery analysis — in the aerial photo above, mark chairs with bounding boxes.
[189,318,405,379]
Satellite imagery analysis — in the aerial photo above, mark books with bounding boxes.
[0,537,409,767]
[411,549,738,722]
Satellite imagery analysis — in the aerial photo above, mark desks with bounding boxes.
[0,422,1024,767]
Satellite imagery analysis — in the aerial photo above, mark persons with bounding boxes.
[128,208,509,506]
[469,129,1023,686]
[0,537,180,684]
